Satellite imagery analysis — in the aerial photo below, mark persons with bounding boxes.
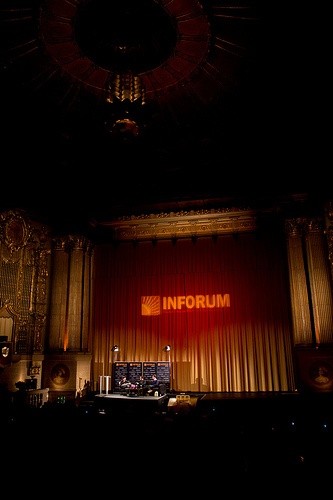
[121,374,160,395]
